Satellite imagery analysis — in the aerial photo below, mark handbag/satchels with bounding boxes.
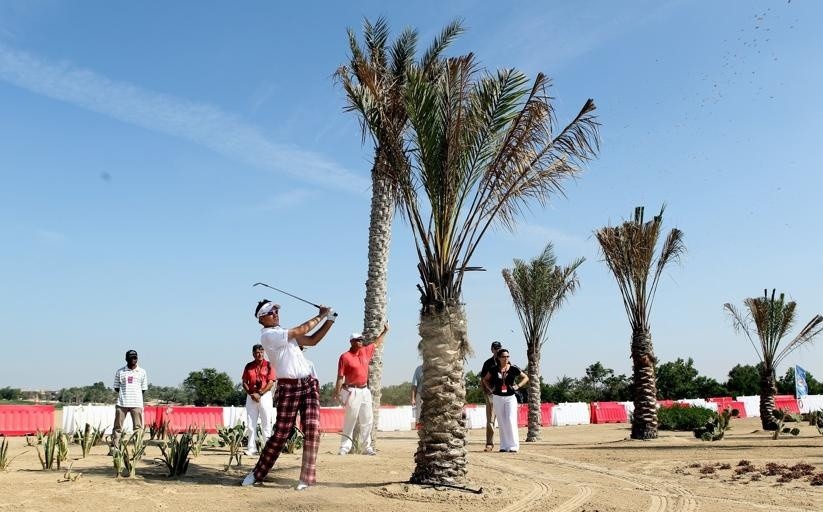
[514,388,524,404]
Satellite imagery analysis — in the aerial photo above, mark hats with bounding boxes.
[349,333,365,342]
[125,349,138,356]
[257,301,281,318]
[492,341,501,347]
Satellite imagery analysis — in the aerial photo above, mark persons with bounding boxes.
[479,341,512,452]
[105,349,147,456]
[332,320,391,456]
[241,344,276,457]
[482,348,530,453]
[240,299,337,490]
[408,361,423,435]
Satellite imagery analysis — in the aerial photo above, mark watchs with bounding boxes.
[257,391,263,395]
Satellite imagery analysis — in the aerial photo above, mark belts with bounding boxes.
[278,375,311,385]
[345,385,367,388]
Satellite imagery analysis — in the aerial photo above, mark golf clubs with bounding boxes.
[252,282,338,317]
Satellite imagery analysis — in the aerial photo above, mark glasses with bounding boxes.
[499,355,510,358]
[126,356,137,360]
[492,347,501,350]
[266,309,278,315]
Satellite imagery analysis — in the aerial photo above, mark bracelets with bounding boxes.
[516,384,520,388]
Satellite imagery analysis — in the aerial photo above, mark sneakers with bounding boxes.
[362,451,376,456]
[483,445,494,452]
[244,450,257,455]
[241,472,256,486]
[106,444,119,456]
[295,479,308,490]
[337,448,347,455]
[499,449,517,453]
